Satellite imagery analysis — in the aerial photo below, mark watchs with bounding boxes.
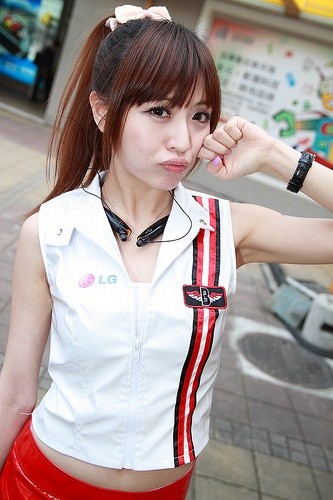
[279,145,317,196]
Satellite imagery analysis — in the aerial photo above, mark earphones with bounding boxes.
[119,228,127,242]
[136,239,148,246]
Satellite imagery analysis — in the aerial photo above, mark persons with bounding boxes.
[0,2,333,498]
[3,11,34,65]
[25,30,63,108]
